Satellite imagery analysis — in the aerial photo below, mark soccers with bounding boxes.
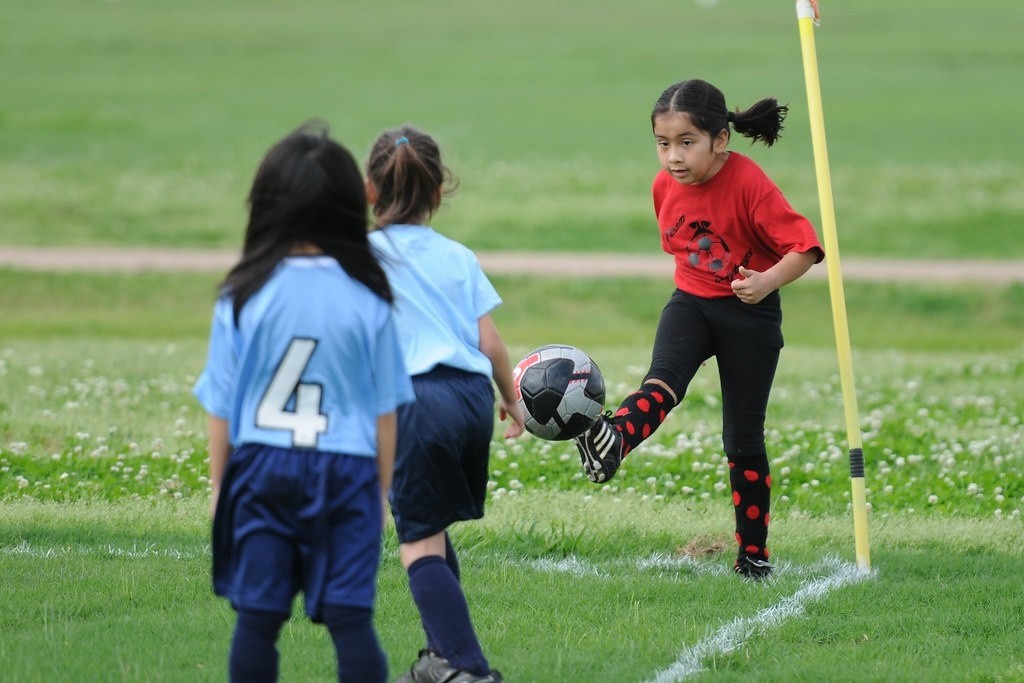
[512,343,608,442]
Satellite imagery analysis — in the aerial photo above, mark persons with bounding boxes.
[364,127,524,683]
[192,131,415,683]
[574,80,825,583]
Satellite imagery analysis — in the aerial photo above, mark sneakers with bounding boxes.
[568,410,623,484]
[392,649,504,683]
[734,550,773,582]
